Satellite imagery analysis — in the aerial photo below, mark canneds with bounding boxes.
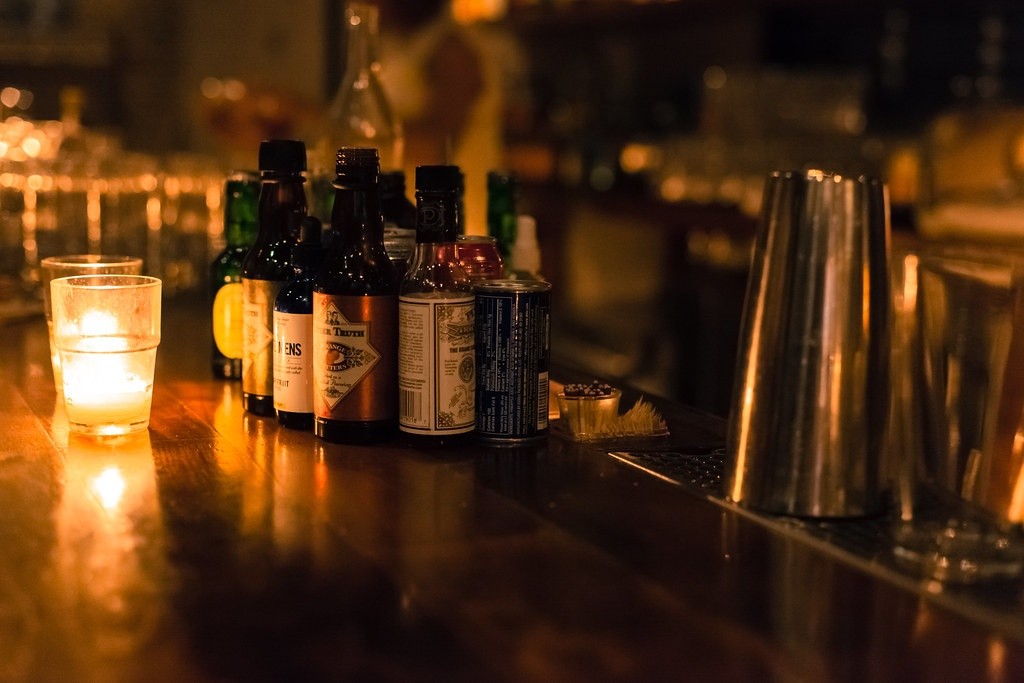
[473,279,552,447]
[432,234,503,286]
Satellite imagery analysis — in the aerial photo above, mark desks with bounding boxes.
[0,286,1024,683]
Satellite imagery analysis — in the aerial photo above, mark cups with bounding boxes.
[896,253,1024,581]
[40,254,144,397]
[48,275,161,435]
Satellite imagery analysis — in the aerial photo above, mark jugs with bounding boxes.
[720,166,897,519]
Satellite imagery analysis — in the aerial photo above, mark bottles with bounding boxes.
[273,218,326,427]
[395,164,479,449]
[209,172,261,379]
[313,149,399,436]
[240,140,307,413]
[318,3,395,178]
